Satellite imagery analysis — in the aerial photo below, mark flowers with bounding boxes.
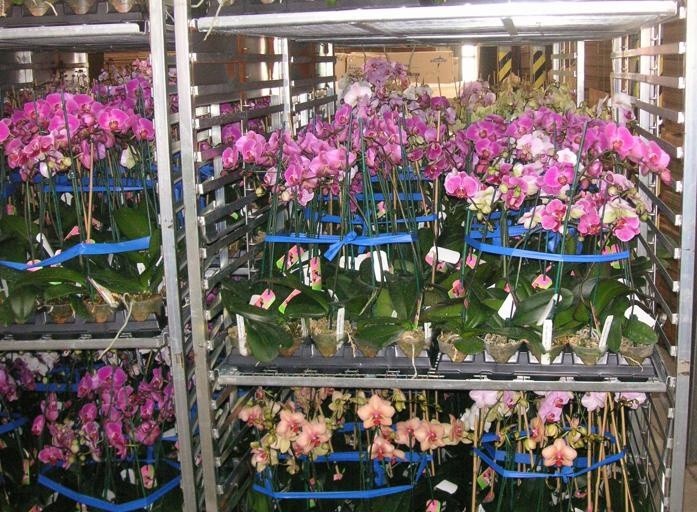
[1,348,184,512]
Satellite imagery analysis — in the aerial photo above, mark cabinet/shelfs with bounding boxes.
[175,0,687,510]
[0,0,196,508]
